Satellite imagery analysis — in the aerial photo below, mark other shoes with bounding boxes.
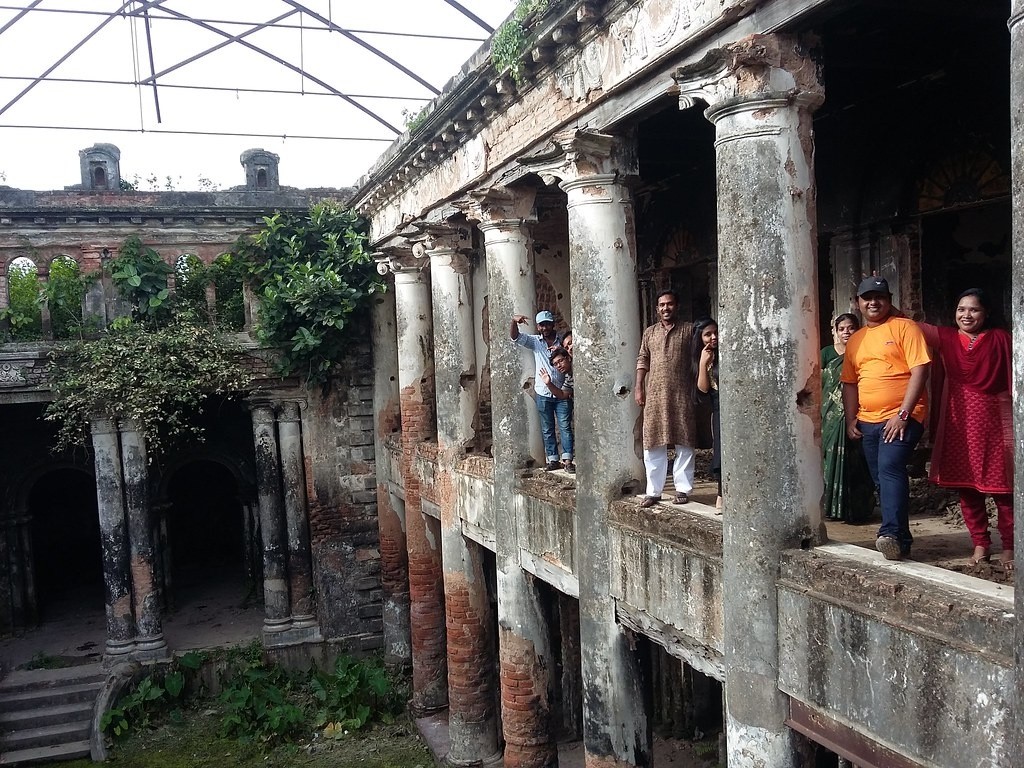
[999,560,1014,570]
[564,463,576,474]
[672,491,688,504]
[639,495,661,508]
[876,535,900,560]
[544,461,562,471]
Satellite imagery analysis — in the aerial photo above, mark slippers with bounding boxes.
[969,556,987,566]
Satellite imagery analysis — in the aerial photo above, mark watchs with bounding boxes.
[897,410,911,420]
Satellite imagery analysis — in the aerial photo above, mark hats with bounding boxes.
[857,276,891,296]
[536,311,553,323]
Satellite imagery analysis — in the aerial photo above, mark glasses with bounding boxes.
[553,357,568,366]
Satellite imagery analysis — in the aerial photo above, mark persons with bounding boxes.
[509,310,577,473]
[546,331,574,359]
[861,270,1014,572]
[539,348,575,399]
[634,289,697,507]
[690,316,723,515]
[820,314,877,528]
[840,276,933,560]
